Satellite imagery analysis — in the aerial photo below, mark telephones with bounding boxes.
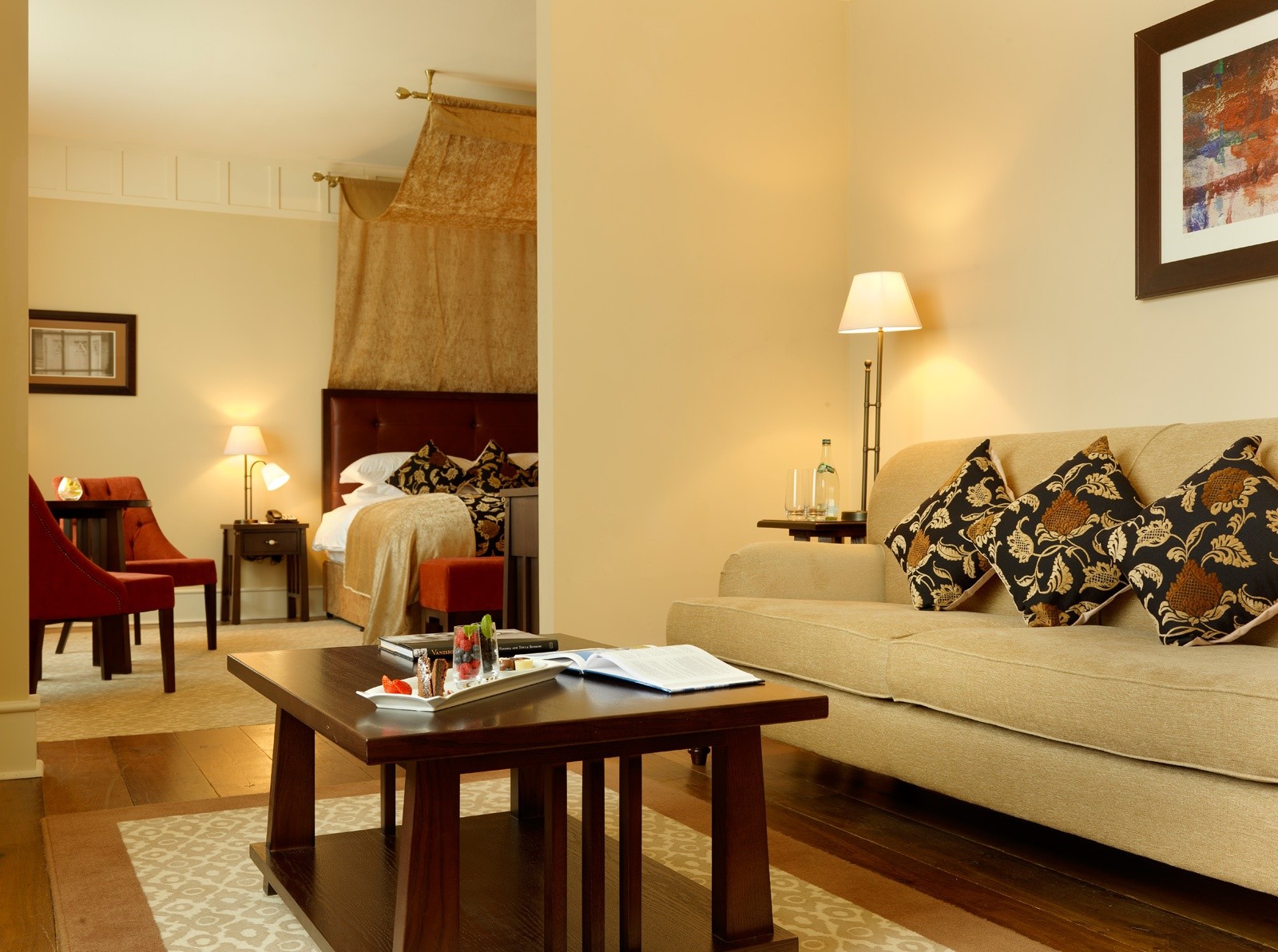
[267,509,299,524]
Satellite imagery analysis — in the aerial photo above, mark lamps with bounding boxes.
[838,271,922,522]
[223,425,290,524]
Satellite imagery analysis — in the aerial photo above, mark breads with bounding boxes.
[417,656,433,698]
[430,659,448,696]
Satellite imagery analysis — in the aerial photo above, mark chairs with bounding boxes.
[53,477,217,654]
[27,473,176,694]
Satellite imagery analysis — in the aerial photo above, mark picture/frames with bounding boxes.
[29,309,136,396]
[1134,0,1278,299]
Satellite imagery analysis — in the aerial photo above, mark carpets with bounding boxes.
[39,760,1060,952]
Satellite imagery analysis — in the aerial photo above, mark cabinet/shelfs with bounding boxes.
[500,488,540,637]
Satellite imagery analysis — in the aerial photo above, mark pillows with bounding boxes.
[339,439,538,507]
[884,435,1278,646]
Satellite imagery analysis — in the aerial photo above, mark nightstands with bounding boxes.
[220,524,310,625]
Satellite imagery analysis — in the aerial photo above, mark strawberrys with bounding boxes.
[381,675,413,695]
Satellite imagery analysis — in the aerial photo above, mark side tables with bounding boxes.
[757,520,867,544]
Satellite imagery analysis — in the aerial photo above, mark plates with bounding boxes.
[501,656,547,677]
[356,658,574,712]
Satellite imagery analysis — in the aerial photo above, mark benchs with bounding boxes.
[419,556,504,633]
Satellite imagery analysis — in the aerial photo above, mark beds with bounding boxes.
[312,389,538,644]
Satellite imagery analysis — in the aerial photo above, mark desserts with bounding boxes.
[500,657,533,671]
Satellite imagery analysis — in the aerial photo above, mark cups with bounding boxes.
[803,466,826,522]
[453,625,484,690]
[471,622,501,682]
[785,467,803,521]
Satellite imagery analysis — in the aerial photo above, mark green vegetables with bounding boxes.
[462,623,478,640]
[480,614,493,642]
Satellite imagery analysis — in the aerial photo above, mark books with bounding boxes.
[514,644,765,693]
[378,629,559,661]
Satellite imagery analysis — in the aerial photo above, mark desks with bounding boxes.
[46,500,153,675]
[227,633,828,952]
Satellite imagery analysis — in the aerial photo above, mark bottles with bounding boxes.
[813,439,839,521]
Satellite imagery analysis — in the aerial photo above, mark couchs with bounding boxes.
[668,418,1278,897]
